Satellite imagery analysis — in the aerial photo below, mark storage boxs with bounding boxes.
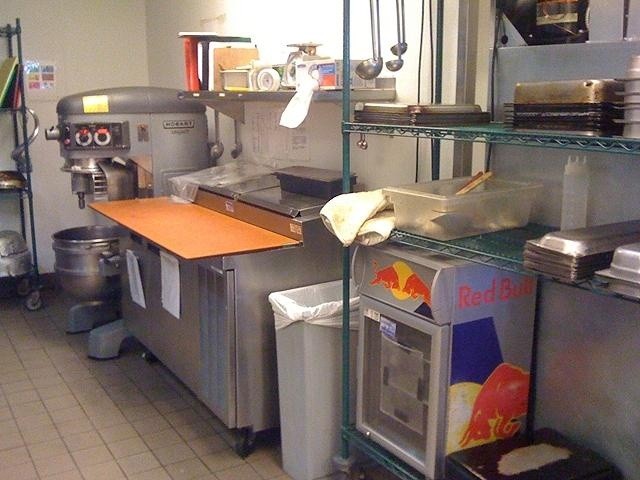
[443,427,617,480]
[382,176,545,243]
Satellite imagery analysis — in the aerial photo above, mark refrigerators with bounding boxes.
[352,239,539,480]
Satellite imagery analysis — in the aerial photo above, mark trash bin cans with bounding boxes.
[268,278,359,480]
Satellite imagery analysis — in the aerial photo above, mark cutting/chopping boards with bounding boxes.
[211,45,259,93]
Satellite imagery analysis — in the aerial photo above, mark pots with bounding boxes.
[0,171,26,192]
[0,228,36,280]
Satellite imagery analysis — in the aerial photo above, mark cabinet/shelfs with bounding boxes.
[0,18,44,310]
[333,0,640,480]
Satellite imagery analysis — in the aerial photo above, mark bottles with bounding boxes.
[562,156,588,231]
